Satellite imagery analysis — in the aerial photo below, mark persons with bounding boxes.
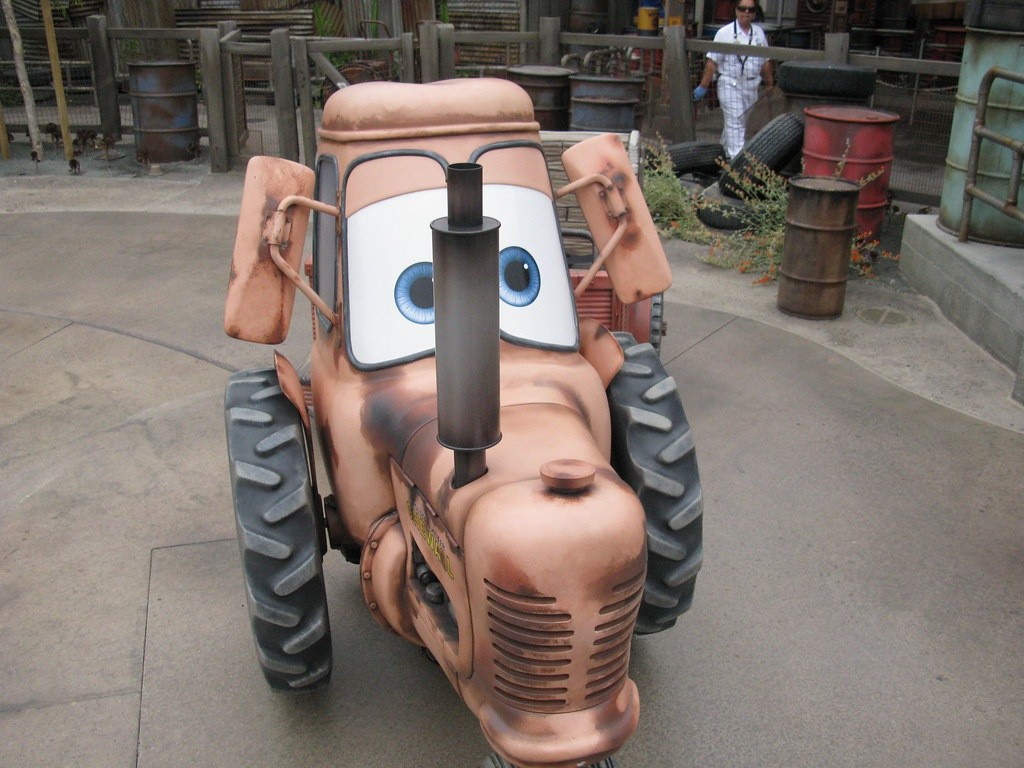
[692,0,775,162]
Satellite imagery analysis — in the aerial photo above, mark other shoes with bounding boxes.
[714,153,730,169]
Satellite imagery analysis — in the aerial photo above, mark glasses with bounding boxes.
[737,5,756,13]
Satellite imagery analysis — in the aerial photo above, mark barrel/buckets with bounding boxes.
[506,65,578,131]
[568,74,645,134]
[127,59,200,164]
[936,26,1024,248]
[801,105,900,266]
[777,175,861,321]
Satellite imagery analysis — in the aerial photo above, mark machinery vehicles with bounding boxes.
[222,80,711,768]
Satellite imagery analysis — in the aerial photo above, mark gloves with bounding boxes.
[692,85,707,101]
